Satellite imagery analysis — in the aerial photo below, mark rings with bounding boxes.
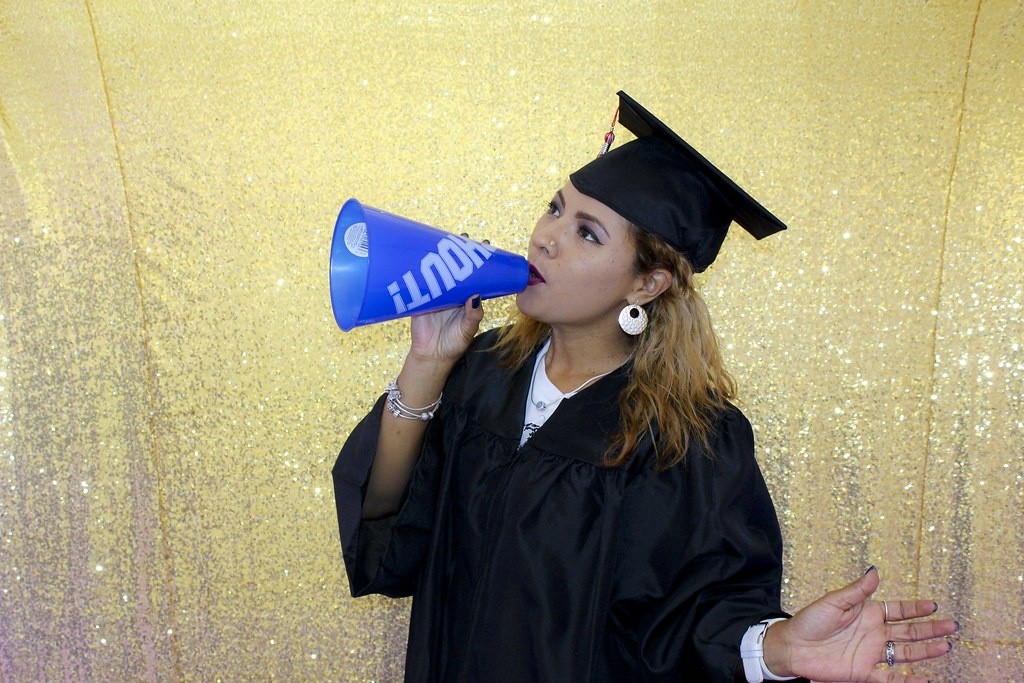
[882,599,889,622]
[886,642,895,666]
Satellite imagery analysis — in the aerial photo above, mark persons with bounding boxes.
[330,91,959,683]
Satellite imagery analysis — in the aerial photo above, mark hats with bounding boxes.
[569,91,787,274]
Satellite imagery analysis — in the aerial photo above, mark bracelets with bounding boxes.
[385,378,443,421]
[740,617,797,683]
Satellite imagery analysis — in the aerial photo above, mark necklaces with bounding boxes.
[529,347,636,410]
